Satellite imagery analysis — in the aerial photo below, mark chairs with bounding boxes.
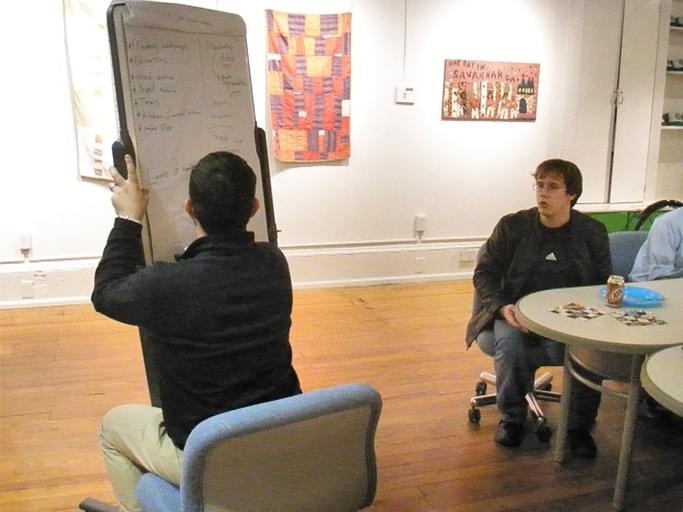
[135,382,382,512]
[469,230,673,441]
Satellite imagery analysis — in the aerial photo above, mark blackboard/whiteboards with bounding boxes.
[107,0,278,265]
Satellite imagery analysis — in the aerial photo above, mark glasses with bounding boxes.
[533,183,566,194]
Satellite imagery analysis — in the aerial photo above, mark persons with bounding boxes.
[91,150,303,511]
[465,159,613,458]
[625,202,683,284]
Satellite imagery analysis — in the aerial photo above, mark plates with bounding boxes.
[602,285,667,305]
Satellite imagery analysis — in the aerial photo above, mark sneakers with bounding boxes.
[569,430,598,457]
[494,415,526,446]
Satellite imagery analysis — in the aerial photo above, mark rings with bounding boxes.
[110,183,118,190]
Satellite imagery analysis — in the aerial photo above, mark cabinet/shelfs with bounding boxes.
[659,24,683,129]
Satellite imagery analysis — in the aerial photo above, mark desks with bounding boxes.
[510,268,683,512]
